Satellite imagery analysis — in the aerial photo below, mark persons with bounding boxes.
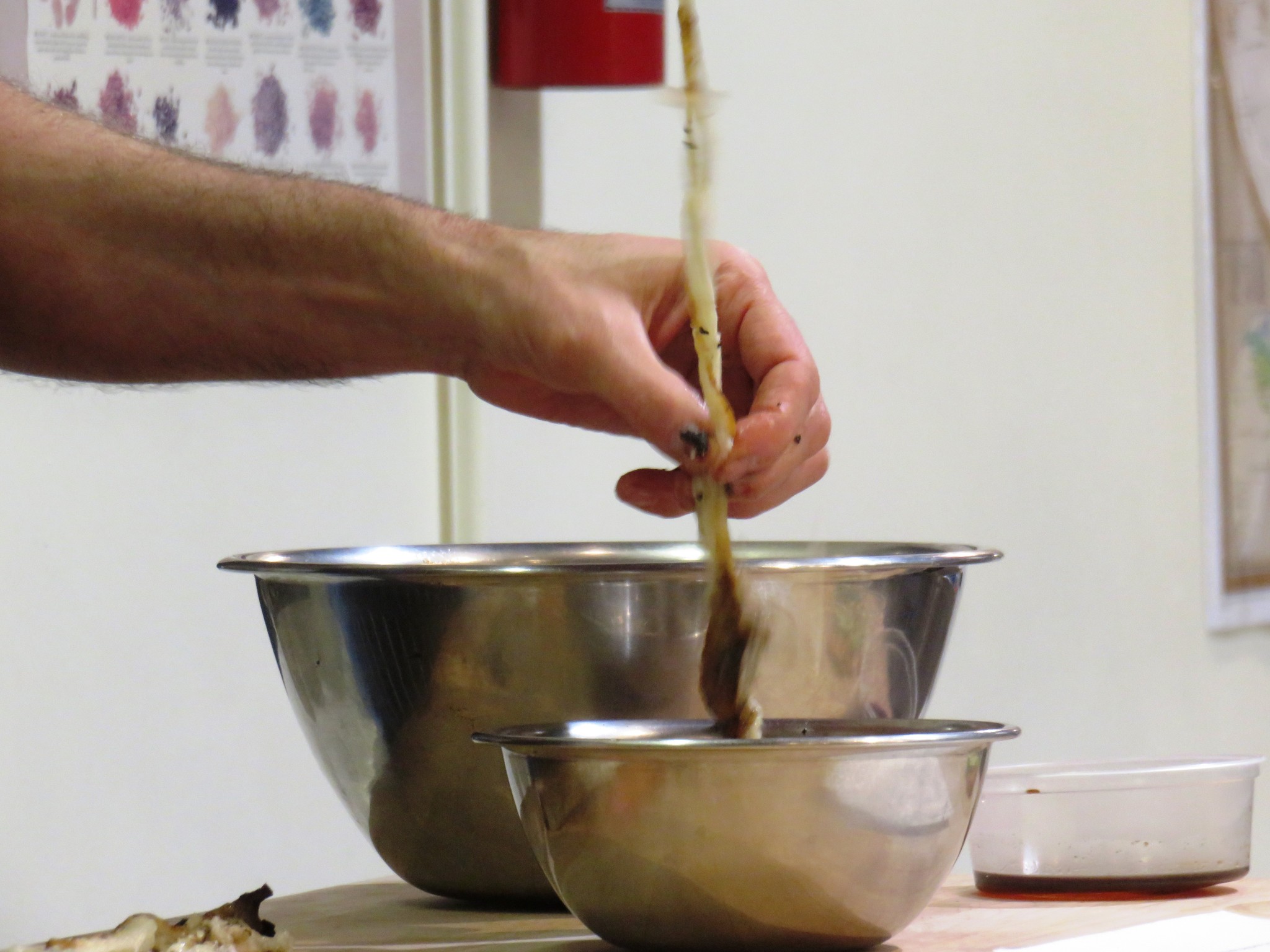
[0,93,831,517]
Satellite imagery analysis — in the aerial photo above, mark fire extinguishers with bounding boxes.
[494,0,665,90]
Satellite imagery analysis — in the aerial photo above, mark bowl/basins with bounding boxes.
[469,715,1025,951]
[966,755,1263,905]
[214,540,1007,913]
